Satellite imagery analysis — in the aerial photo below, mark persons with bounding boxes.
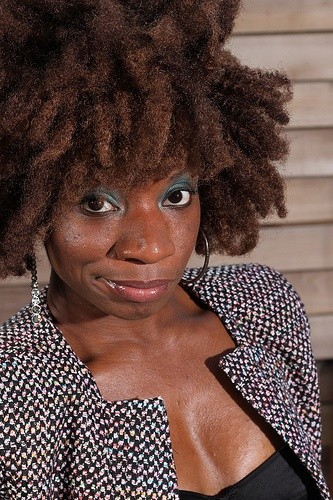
[0,0,330,498]
